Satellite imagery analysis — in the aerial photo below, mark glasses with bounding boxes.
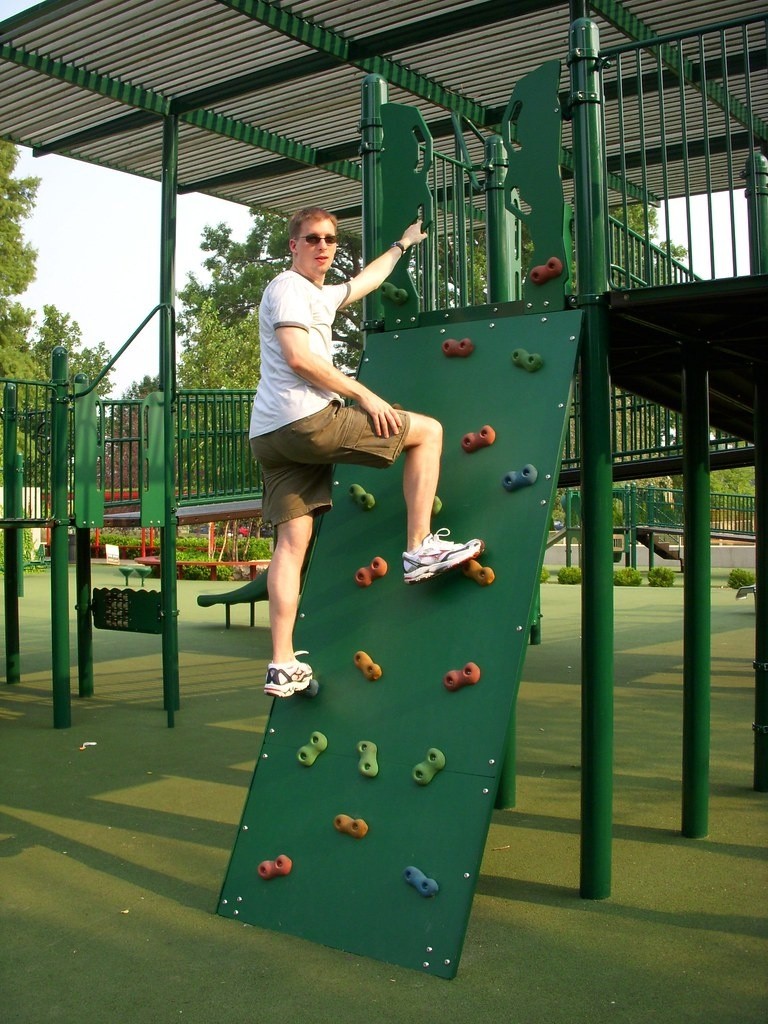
[293,234,338,244]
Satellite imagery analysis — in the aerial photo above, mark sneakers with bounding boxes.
[264,650,313,697]
[403,528,485,584]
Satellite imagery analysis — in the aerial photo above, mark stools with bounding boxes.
[135,566,151,586]
[119,567,134,587]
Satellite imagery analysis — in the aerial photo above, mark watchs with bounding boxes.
[391,241,406,256]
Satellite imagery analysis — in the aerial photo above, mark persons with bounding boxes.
[249,206,488,702]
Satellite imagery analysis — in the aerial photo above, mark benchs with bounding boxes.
[136,555,272,582]
